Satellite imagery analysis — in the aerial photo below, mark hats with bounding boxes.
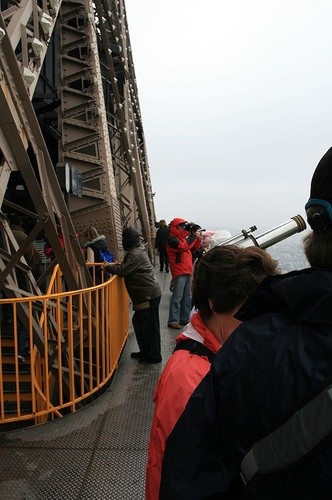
[304,146,332,228]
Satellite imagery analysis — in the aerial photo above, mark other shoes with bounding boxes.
[131,351,144,359]
[139,358,152,364]
[18,354,26,362]
[168,323,181,329]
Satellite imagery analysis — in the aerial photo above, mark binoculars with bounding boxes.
[186,223,201,232]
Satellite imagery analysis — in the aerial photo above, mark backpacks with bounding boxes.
[98,249,113,276]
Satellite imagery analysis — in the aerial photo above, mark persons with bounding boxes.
[144,243,280,500]
[44,232,84,306]
[85,225,117,279]
[154,219,211,328]
[5,214,42,361]
[101,227,163,364]
[157,144,332,500]
